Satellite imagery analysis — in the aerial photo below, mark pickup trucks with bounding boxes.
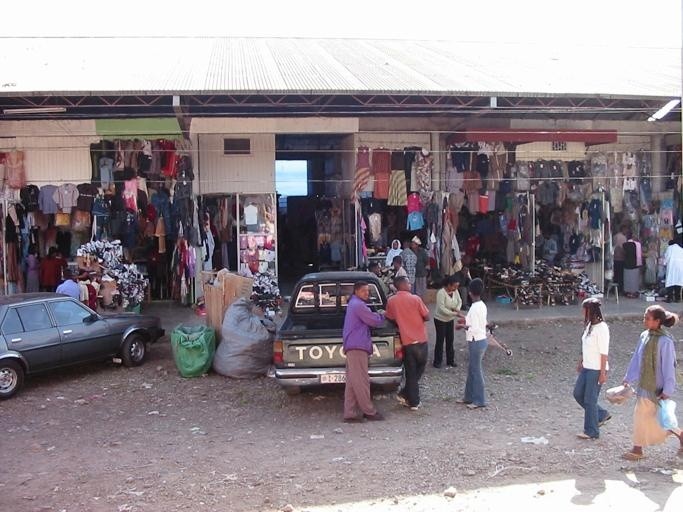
[273,271,406,396]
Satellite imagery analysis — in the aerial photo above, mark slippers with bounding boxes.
[622,451,648,460]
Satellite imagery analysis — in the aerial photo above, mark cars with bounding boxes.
[0,292,165,400]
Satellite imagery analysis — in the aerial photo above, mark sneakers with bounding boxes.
[410,401,422,410]
[395,394,409,407]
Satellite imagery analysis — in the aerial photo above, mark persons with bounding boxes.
[613,225,628,296]
[455,277,489,407]
[392,257,406,277]
[434,276,462,367]
[56,269,81,300]
[39,247,68,292]
[385,239,403,266]
[574,298,612,439]
[369,260,392,288]
[411,236,428,301]
[75,270,97,310]
[400,239,417,295]
[623,304,683,459]
[26,245,41,292]
[664,232,683,302]
[455,309,514,357]
[377,275,432,410]
[342,281,387,423]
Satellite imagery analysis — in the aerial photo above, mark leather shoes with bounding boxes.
[456,399,464,403]
[576,433,590,439]
[363,412,384,421]
[343,416,366,423]
[467,403,478,408]
[597,415,612,427]
[433,360,442,368]
[447,362,457,367]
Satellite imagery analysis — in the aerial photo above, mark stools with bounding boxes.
[605,282,621,305]
